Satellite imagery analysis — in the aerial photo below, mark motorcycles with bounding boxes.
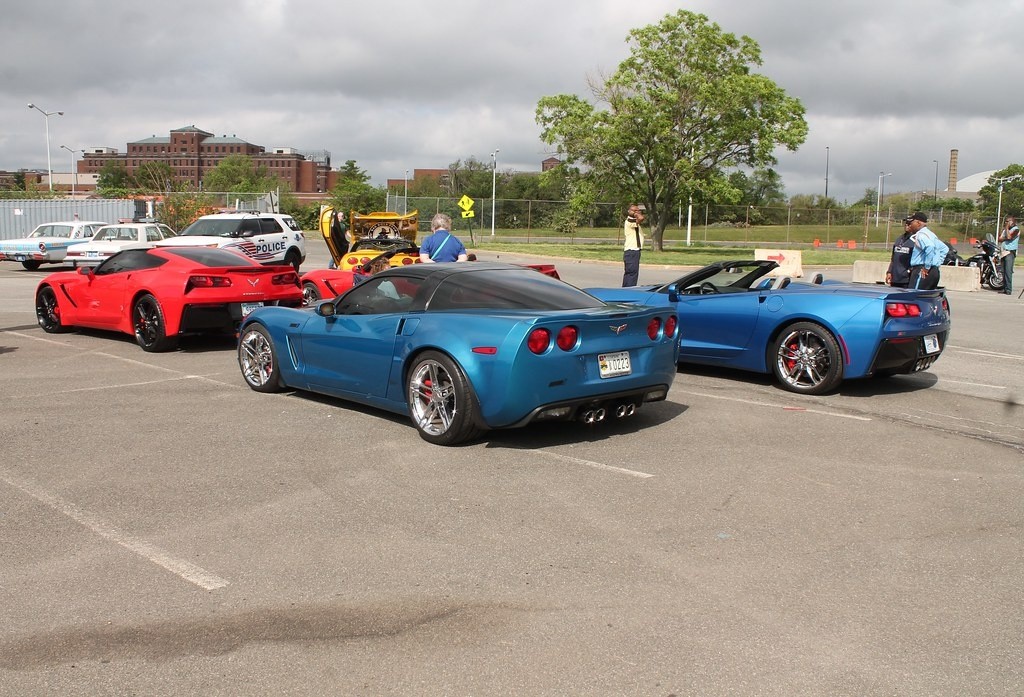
[943,233,1004,290]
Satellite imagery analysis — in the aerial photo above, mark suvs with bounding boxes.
[154,213,307,274]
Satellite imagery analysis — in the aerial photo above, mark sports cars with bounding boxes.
[319,205,423,273]
[581,261,954,396]
[35,246,305,353]
[298,250,560,314]
[237,260,685,447]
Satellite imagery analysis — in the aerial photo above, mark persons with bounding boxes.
[370,257,399,299]
[903,211,949,289]
[622,206,645,288]
[328,213,351,270]
[885,217,915,288]
[419,213,468,263]
[997,215,1020,294]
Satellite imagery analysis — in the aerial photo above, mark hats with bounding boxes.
[903,216,913,221]
[907,212,927,222]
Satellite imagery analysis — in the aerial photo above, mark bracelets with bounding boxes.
[633,213,635,217]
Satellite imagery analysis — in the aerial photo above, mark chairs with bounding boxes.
[770,275,791,290]
[809,271,823,285]
[119,235,132,240]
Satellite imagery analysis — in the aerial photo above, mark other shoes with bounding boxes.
[1005,290,1012,295]
[329,267,336,269]
[999,289,1006,293]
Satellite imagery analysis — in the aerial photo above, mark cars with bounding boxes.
[0,221,110,271]
[63,222,177,272]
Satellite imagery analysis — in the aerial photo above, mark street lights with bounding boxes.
[825,146,829,198]
[61,145,85,200]
[876,171,892,227]
[933,160,938,200]
[405,171,408,216]
[28,104,64,192]
[490,149,499,236]
[985,174,1022,247]
